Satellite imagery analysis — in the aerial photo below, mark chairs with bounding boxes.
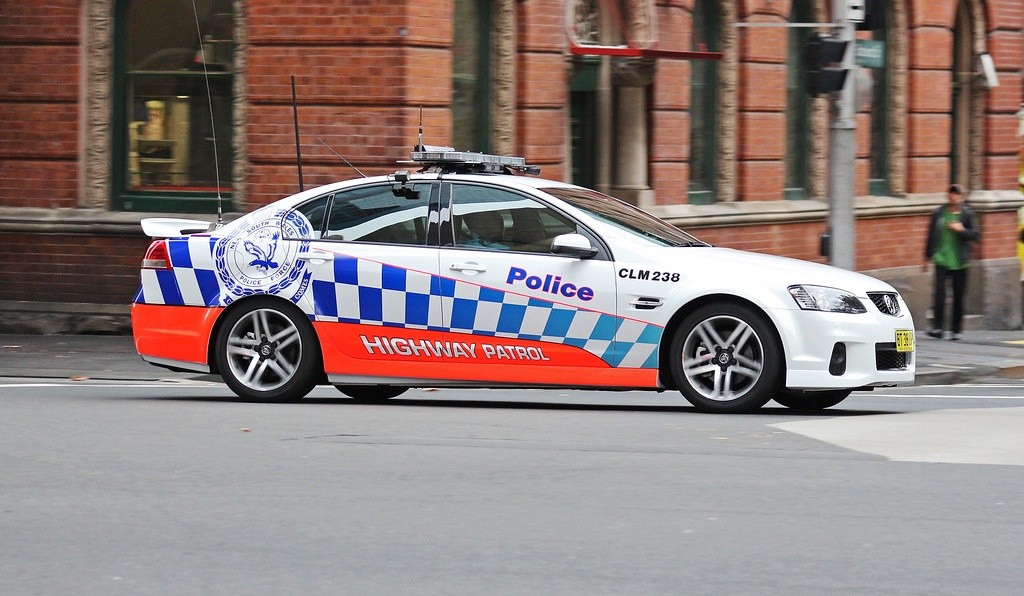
[464,207,552,247]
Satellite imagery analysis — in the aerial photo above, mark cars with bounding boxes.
[131,141,915,413]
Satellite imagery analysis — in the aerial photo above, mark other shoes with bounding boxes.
[952,333,960,340]
[927,329,942,338]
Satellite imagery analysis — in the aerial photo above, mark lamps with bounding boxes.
[952,55,1000,91]
[195,33,234,64]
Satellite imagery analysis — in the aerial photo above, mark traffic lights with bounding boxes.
[808,32,851,96]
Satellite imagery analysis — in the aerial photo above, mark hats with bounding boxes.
[949,184,965,195]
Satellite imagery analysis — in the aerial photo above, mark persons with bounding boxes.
[925,184,979,339]
[462,208,576,251]
